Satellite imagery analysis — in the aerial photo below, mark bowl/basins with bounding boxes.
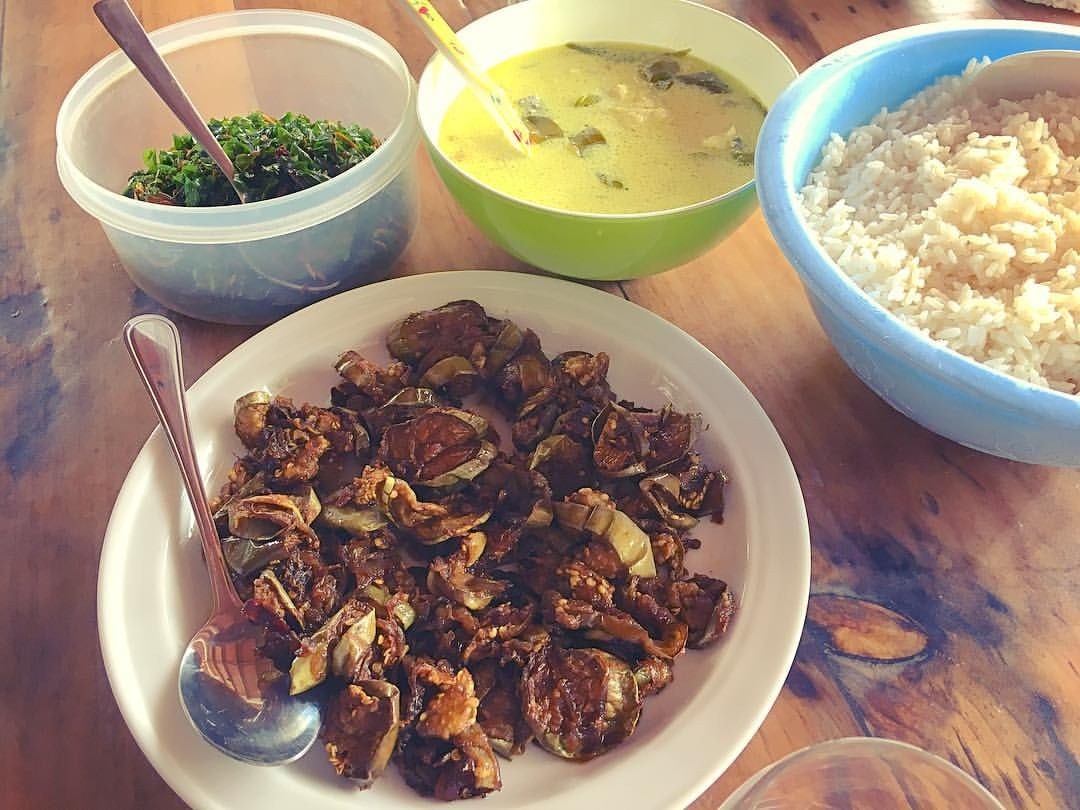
[416,0,800,281]
[55,9,419,326]
[754,18,1080,468]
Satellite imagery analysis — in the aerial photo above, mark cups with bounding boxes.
[717,736,1008,810]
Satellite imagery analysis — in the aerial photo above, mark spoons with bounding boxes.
[123,315,321,764]
[92,1,342,291]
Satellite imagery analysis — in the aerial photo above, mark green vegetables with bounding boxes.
[121,110,389,282]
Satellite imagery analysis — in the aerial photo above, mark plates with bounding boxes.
[97,274,811,810]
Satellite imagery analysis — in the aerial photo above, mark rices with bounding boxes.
[797,54,1080,403]
